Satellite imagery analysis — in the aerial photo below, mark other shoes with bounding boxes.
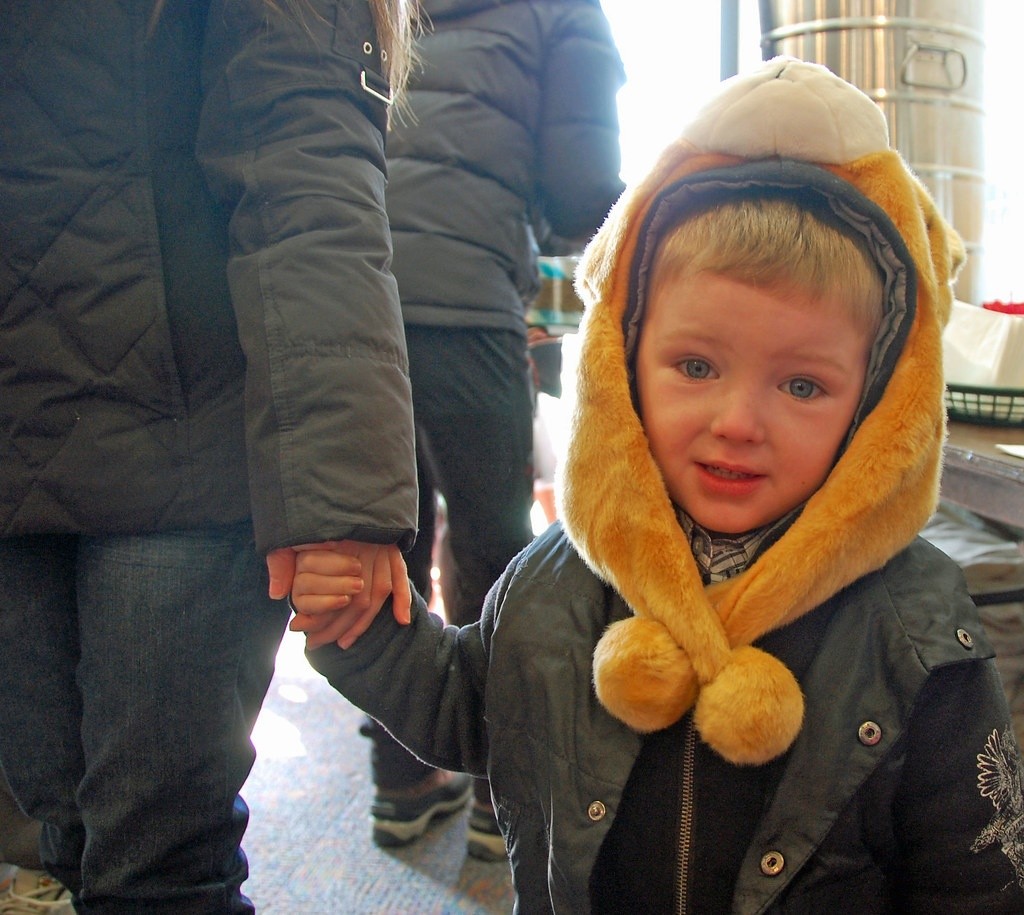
[357,770,471,848]
[466,807,506,863]
[0,868,72,915]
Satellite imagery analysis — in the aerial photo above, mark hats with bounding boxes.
[557,53,966,761]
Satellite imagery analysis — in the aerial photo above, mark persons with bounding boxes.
[0,0,419,915]
[292,56,1024,915]
[360,1,626,858]
